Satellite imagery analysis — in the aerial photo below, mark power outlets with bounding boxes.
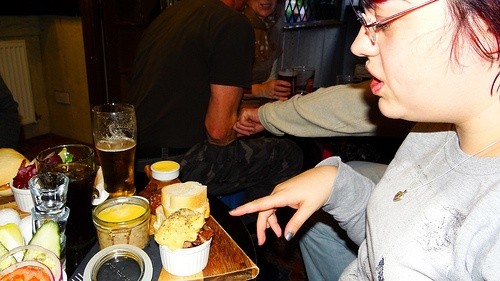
[53,90,71,107]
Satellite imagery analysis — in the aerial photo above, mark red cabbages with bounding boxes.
[13,159,37,190]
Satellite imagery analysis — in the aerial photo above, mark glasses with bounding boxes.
[357,0,439,42]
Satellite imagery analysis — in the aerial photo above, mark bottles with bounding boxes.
[140,162,182,214]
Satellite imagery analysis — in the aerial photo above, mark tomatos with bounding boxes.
[0,266,52,281]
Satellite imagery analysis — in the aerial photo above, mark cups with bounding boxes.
[92,195,152,260]
[158,239,212,277]
[9,178,35,213]
[28,171,69,213]
[276,64,364,98]
[35,143,97,251]
[90,101,138,199]
[31,207,70,251]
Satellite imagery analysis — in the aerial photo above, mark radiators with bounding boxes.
[0,39,43,126]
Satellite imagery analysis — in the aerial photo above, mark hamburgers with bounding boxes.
[0,147,30,196]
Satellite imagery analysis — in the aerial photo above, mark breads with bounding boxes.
[154,208,205,248]
[154,182,210,229]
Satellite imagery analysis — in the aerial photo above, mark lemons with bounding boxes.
[58,147,73,168]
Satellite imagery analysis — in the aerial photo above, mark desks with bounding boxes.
[0,160,260,281]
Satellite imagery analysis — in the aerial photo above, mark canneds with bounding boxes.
[93,197,150,252]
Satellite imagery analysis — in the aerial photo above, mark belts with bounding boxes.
[133,145,190,158]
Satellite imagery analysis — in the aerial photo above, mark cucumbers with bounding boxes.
[20,220,61,278]
[0,242,18,270]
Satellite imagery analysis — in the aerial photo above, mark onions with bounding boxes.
[0,244,63,281]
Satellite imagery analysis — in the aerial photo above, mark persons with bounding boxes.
[0,75,20,155]
[231,77,411,281]
[118,0,301,255]
[224,0,500,281]
[235,0,293,102]
